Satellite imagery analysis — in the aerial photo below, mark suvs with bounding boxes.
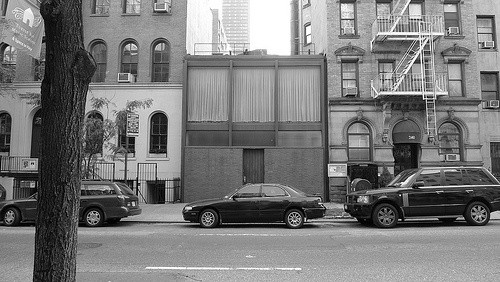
[0,180,142,227]
[343,165,500,228]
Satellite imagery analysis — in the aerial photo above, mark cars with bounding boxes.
[181,182,327,228]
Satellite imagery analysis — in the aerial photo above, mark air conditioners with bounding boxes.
[445,154,460,161]
[153,3,168,12]
[483,41,494,49]
[346,87,357,95]
[488,99,499,108]
[343,27,353,34]
[449,27,459,35]
[118,73,131,83]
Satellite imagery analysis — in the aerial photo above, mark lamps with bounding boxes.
[382,132,388,141]
[429,132,434,142]
